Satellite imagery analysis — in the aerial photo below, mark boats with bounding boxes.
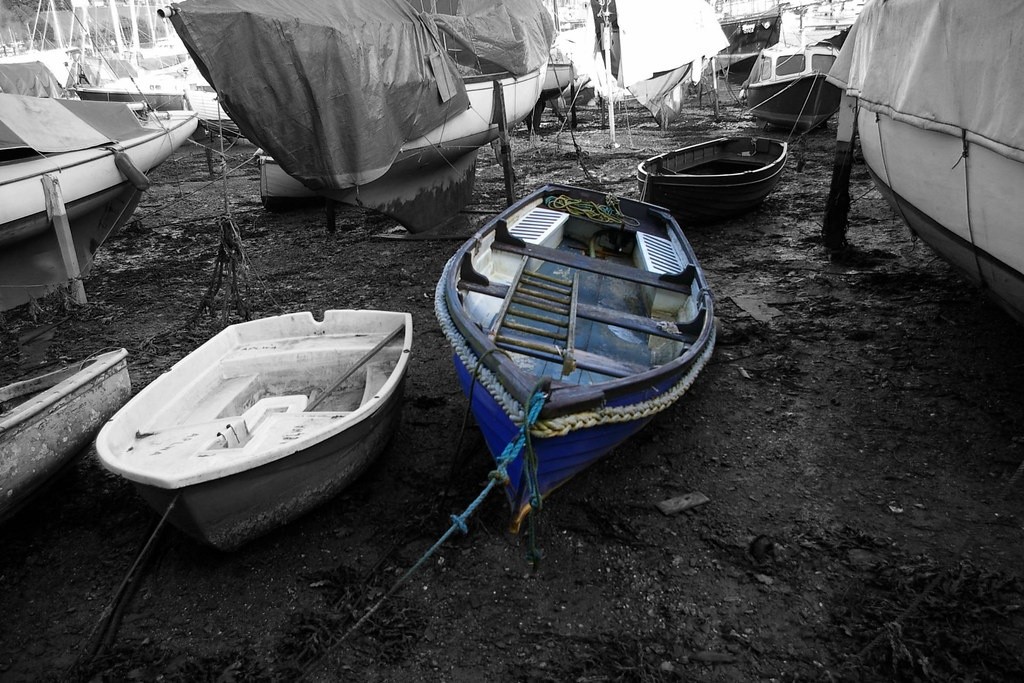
[742,41,843,130]
[0,348,133,523]
[713,0,791,56]
[157,1,575,236]
[636,134,788,225]
[95,310,413,552]
[434,183,717,534]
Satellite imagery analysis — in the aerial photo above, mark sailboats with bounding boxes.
[0,0,249,313]
